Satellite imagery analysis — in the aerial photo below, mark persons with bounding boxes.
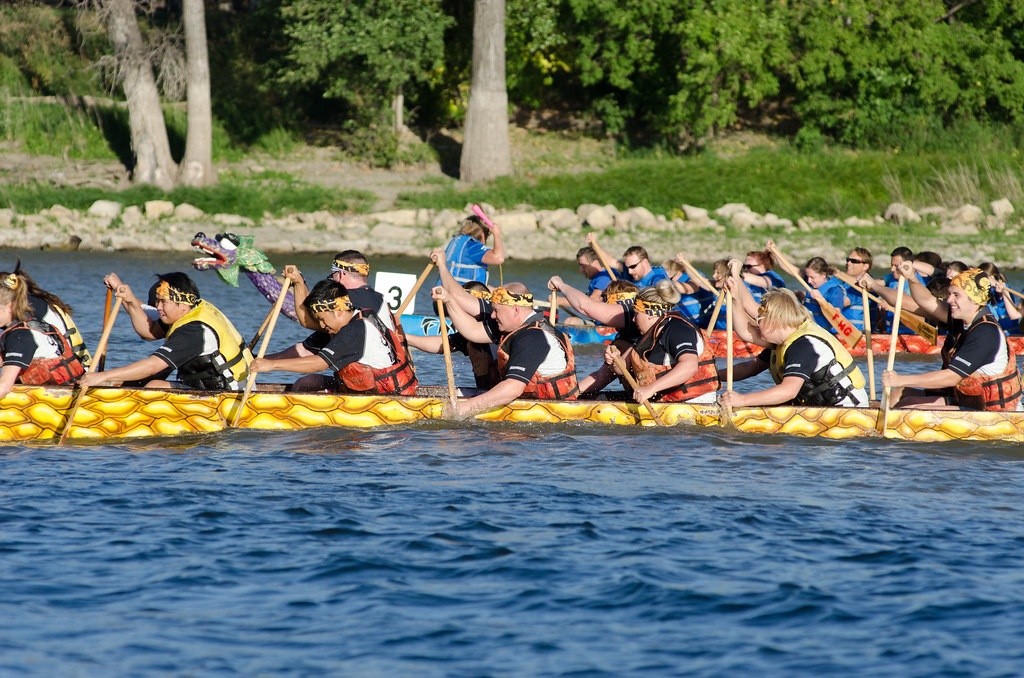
[77,272,256,391]
[716,258,870,409]
[0,271,94,401]
[549,234,1024,337]
[405,280,581,419]
[246,279,420,396]
[857,267,1024,411]
[546,275,722,404]
[248,249,416,393]
[433,213,505,317]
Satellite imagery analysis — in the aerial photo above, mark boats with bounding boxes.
[189,229,1024,359]
[0,384,1024,446]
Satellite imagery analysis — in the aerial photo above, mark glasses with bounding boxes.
[626,259,643,269]
[743,264,760,270]
[846,257,866,263]
[327,270,346,281]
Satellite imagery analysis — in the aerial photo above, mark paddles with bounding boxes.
[56,286,128,445]
[589,233,617,281]
[725,280,733,417]
[609,345,664,426]
[229,267,294,426]
[875,265,910,435]
[247,278,293,352]
[678,253,726,302]
[768,243,864,350]
[835,270,938,347]
[861,280,876,400]
[549,281,557,326]
[98,285,113,372]
[395,252,438,319]
[706,283,726,338]
[435,288,459,407]
[998,280,1024,299]
